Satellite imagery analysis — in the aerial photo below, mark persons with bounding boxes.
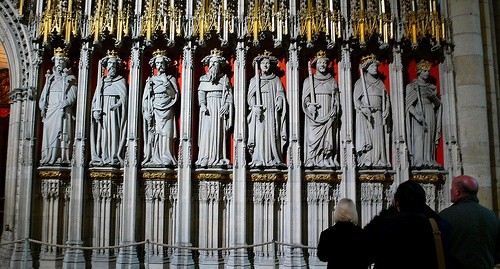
[365,180,445,269]
[301,50,342,168]
[355,55,392,168]
[404,60,444,168]
[195,48,231,168]
[90,49,128,166]
[141,49,179,167]
[439,176,500,269]
[38,48,77,165]
[245,50,287,166]
[317,198,365,269]
